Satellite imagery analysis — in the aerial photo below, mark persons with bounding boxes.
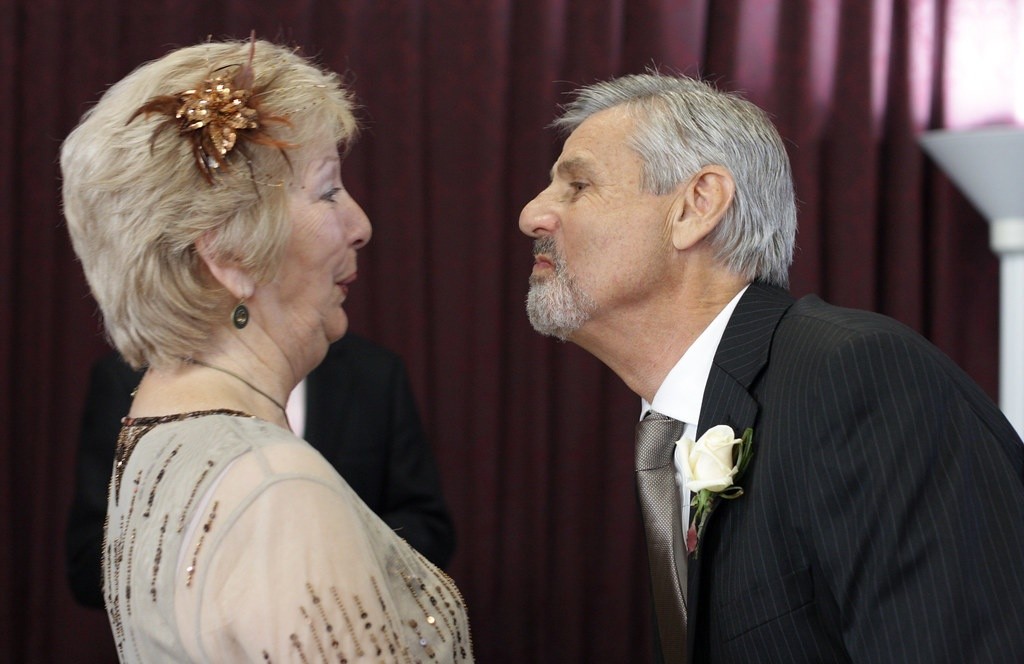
[518,74,1024,663]
[59,41,473,664]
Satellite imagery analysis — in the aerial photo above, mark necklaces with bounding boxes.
[172,352,291,431]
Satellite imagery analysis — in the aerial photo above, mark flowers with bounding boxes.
[675,425,754,561]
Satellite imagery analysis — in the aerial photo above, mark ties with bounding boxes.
[634,411,687,664]
